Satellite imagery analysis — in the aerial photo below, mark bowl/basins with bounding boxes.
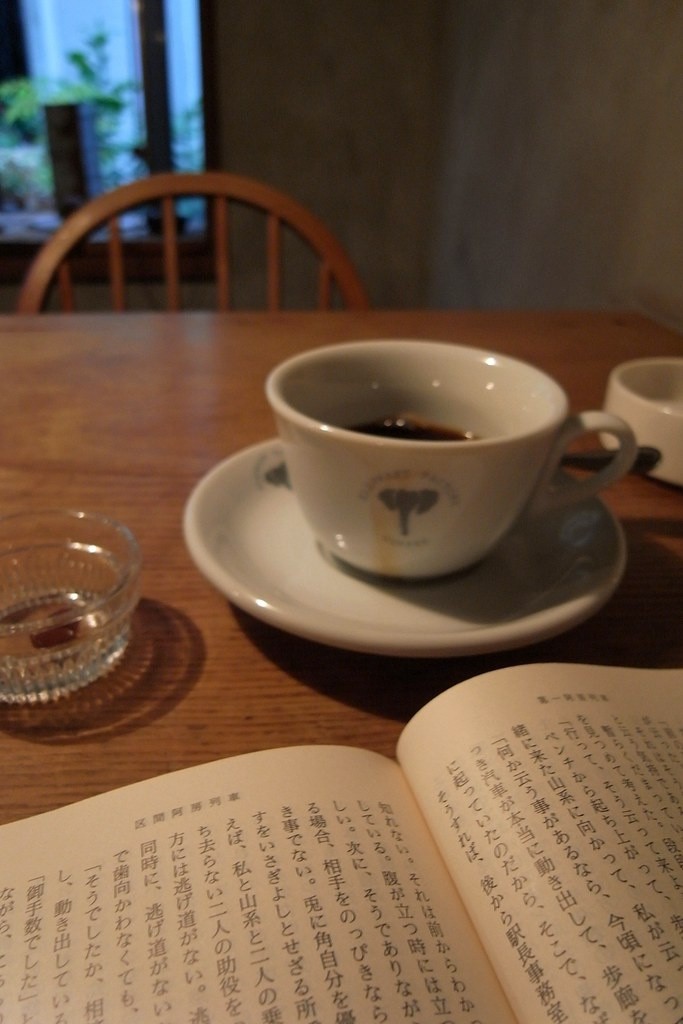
[0,508,145,703]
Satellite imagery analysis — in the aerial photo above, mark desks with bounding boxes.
[0,309,683,825]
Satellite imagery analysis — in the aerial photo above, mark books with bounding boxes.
[0,660,683,1024]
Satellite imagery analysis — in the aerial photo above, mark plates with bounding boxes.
[183,437,628,659]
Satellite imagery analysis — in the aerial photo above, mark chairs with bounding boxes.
[15,172,373,313]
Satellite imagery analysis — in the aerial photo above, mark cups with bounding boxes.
[264,339,638,577]
[600,355,683,485]
